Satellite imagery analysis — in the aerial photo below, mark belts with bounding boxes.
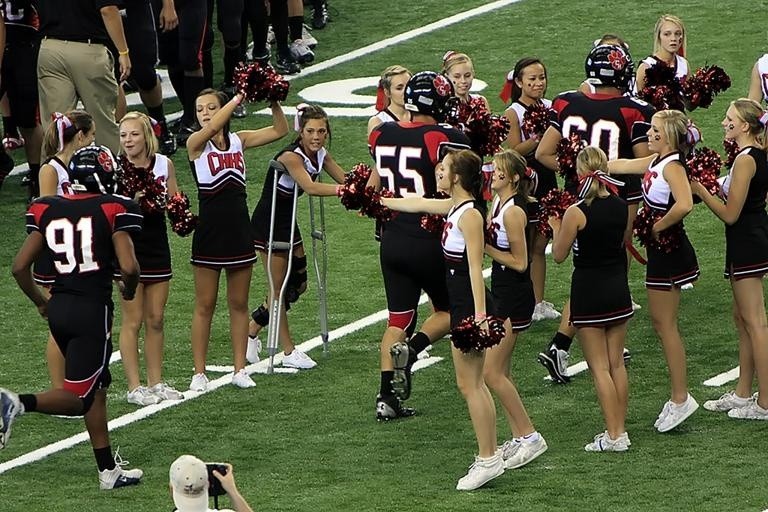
[40,31,110,46]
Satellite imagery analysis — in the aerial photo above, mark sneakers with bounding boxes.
[310,0,330,30]
[97,457,144,490]
[702,390,753,414]
[535,342,570,385]
[125,385,161,406]
[189,373,209,392]
[454,451,506,492]
[727,397,768,422]
[652,392,699,434]
[145,383,185,401]
[281,347,319,369]
[584,429,631,453]
[388,342,419,402]
[497,430,549,472]
[156,115,203,158]
[374,393,416,420]
[231,368,257,389]
[244,335,262,364]
[247,21,318,77]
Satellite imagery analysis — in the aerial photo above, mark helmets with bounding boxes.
[67,145,118,196]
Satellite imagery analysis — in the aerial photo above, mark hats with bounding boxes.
[1,387,26,451]
[167,453,210,512]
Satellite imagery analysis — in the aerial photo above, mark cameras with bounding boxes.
[206,462,227,498]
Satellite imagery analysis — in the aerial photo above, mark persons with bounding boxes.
[373,148,505,492]
[636,108,701,433]
[0,144,143,491]
[547,146,631,452]
[482,147,547,471]
[165,454,250,512]
